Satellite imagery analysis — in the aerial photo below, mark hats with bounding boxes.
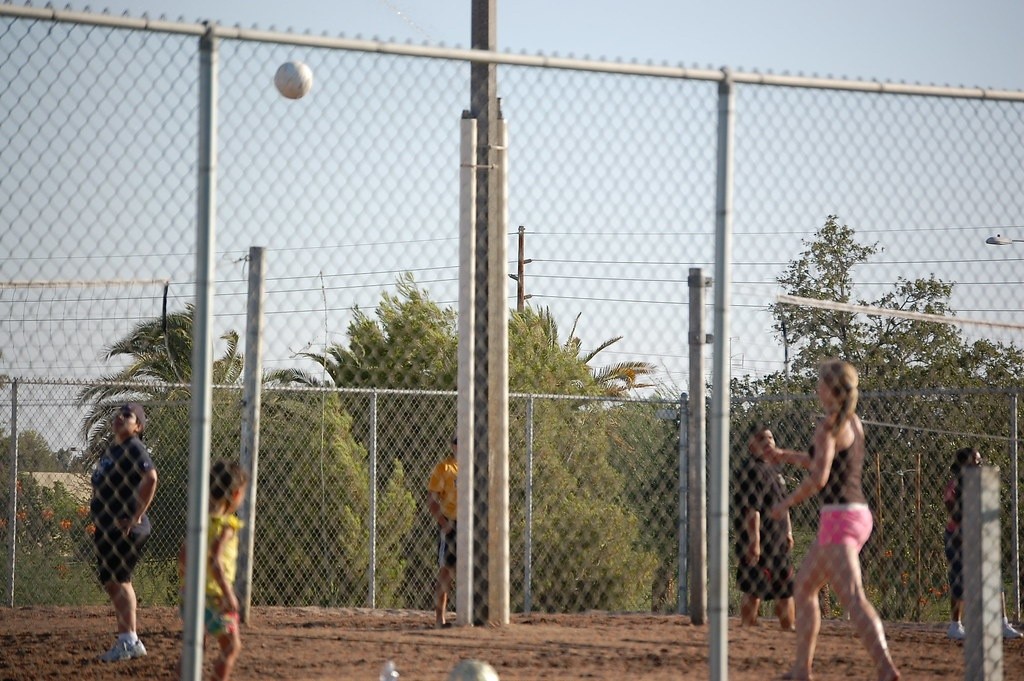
[121,404,146,432]
[949,446,982,474]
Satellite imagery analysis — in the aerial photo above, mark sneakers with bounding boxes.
[1002,623,1023,639]
[947,624,965,640]
[100,640,149,661]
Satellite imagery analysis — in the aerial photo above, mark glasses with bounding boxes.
[451,437,458,446]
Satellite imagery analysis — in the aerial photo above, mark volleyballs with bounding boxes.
[449,659,500,681]
[274,60,313,100]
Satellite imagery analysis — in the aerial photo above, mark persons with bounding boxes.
[177,462,246,681]
[428,436,457,623]
[943,447,1024,640]
[91,404,157,661]
[734,426,795,629]
[765,361,899,681]
